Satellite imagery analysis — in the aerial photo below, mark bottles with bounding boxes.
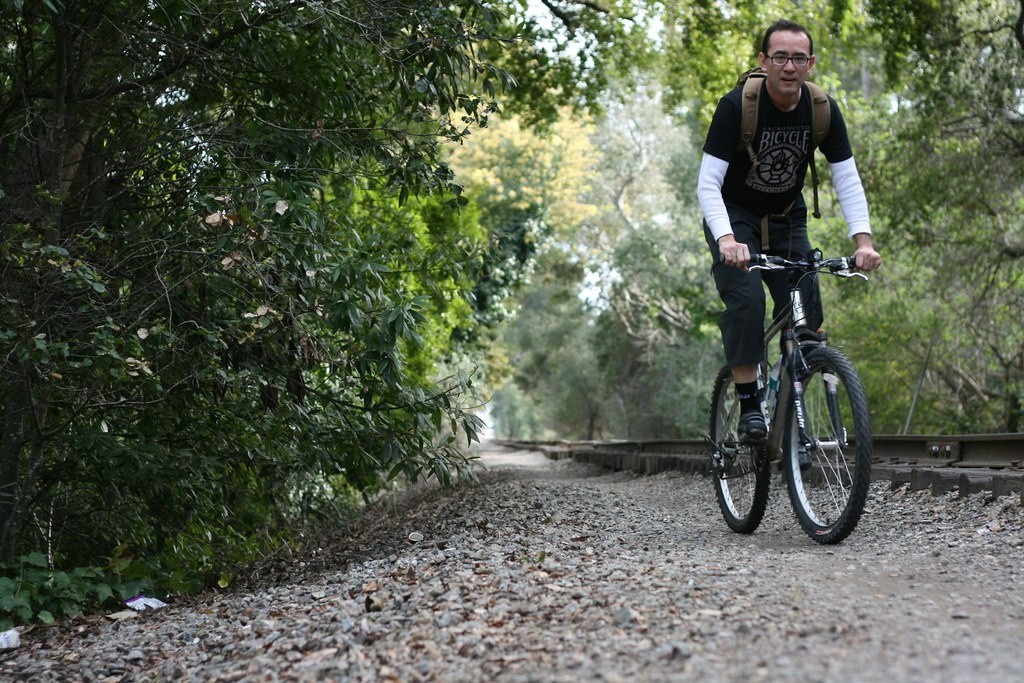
[765,355,784,407]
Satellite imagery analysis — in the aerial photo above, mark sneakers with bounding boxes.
[799,441,812,469]
[737,412,767,442]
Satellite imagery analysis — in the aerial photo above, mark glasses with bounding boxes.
[767,53,812,68]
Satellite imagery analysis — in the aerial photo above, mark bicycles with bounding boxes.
[704,247,875,545]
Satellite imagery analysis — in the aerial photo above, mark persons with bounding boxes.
[697,18,882,475]
